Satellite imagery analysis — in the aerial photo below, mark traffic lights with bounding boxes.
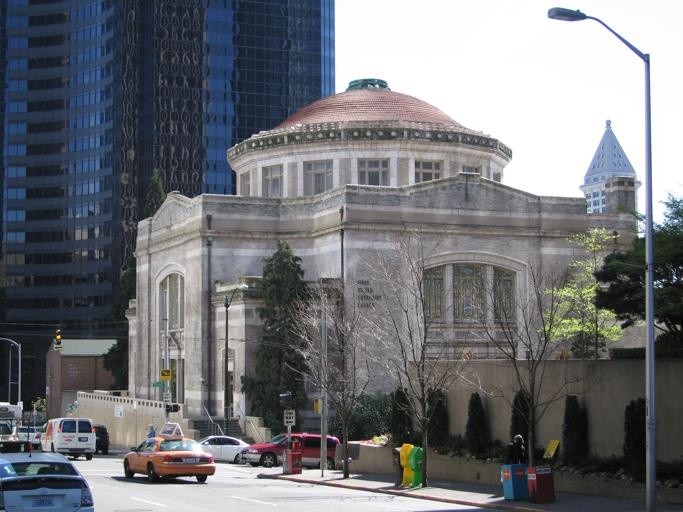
[279,393,290,407]
[54,327,63,345]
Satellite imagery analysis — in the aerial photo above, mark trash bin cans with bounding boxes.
[500,463,555,504]
[283,441,303,474]
[392,443,424,488]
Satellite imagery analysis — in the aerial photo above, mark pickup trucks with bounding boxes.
[1,420,29,453]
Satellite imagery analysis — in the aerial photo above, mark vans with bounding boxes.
[18,418,109,459]
[240,433,340,470]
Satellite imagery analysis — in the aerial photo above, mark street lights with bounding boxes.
[224,283,250,433]
[547,6,659,510]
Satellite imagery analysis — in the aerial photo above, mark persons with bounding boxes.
[145,423,155,438]
[507,434,525,464]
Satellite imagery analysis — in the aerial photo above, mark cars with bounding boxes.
[197,435,251,465]
[124,436,215,483]
[0,452,94,512]
